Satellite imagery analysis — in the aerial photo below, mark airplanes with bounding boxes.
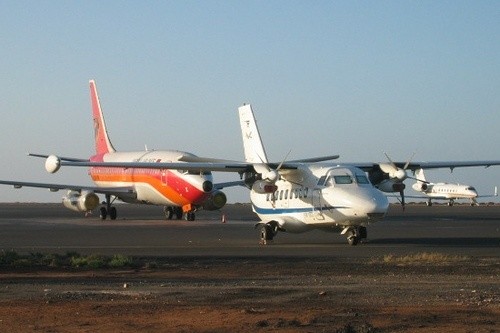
[408,167,480,207]
[0,80,341,223]
[44,100,500,247]
